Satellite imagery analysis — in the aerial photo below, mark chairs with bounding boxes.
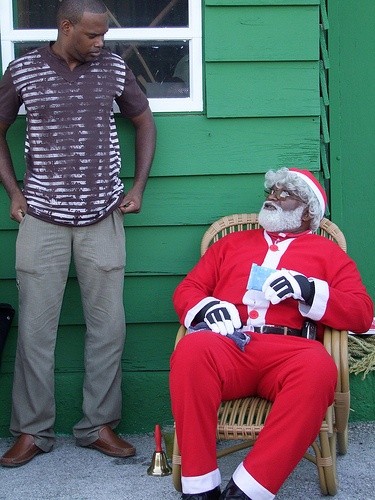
[172,213,350,495]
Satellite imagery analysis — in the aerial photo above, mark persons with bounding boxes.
[170,166,374,500]
[0,0,157,470]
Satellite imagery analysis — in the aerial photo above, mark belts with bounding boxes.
[254,327,302,337]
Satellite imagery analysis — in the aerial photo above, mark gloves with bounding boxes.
[262,267,314,306]
[195,300,242,336]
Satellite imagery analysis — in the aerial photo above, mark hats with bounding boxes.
[286,168,328,220]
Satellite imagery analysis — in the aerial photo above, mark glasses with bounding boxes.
[264,187,306,204]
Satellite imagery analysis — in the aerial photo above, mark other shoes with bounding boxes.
[181,485,221,500]
[221,478,252,500]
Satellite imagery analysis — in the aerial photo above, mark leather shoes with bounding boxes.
[89,425,136,457]
[0,434,43,467]
